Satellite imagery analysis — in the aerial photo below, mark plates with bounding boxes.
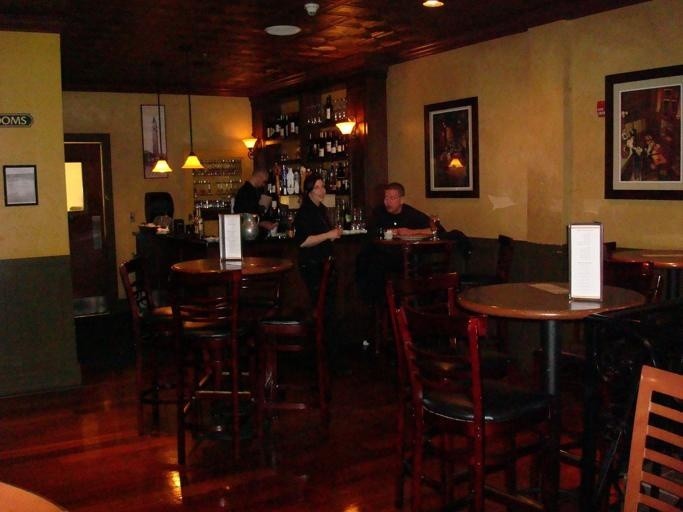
[396,235,425,241]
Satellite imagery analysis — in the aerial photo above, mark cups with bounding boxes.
[305,98,347,125]
[280,152,288,162]
[177,223,183,233]
[185,224,193,234]
[429,215,441,241]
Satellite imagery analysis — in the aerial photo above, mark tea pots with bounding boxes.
[239,214,260,240]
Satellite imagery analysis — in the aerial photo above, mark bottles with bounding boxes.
[265,111,299,139]
[311,130,349,159]
[192,202,203,237]
[270,195,277,216]
[324,95,332,123]
[288,198,366,233]
[268,161,348,194]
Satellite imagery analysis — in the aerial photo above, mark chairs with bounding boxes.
[366,234,682,511]
[120,218,336,491]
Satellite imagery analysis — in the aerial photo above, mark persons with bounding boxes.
[291,173,356,378]
[367,182,443,308]
[232,167,278,236]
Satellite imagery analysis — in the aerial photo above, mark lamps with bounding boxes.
[151,94,173,173]
[181,94,205,169]
[242,138,257,159]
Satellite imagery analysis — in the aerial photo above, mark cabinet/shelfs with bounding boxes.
[249,52,387,227]
[193,160,242,221]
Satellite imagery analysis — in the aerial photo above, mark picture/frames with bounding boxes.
[424,96,479,198]
[3,165,38,206]
[605,64,683,201]
[140,104,168,179]
